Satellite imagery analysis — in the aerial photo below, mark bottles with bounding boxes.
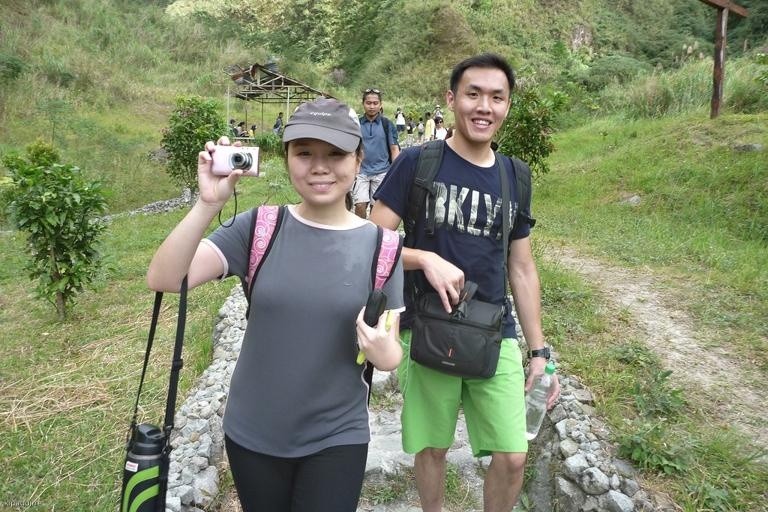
[120,422,169,511]
[524,364,556,441]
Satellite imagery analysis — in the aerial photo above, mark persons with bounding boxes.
[395,102,447,141]
[247,125,257,140]
[228,119,238,137]
[144,97,408,510]
[351,89,402,219]
[276,112,284,127]
[272,123,283,135]
[365,52,563,511]
[235,122,249,139]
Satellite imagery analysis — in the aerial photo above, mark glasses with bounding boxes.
[365,88,381,94]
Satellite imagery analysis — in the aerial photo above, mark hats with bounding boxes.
[281,96,363,152]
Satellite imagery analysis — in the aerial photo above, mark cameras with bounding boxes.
[210,145,261,178]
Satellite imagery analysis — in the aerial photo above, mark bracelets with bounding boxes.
[526,345,552,361]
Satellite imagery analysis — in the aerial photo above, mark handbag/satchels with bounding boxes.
[410,294,505,380]
